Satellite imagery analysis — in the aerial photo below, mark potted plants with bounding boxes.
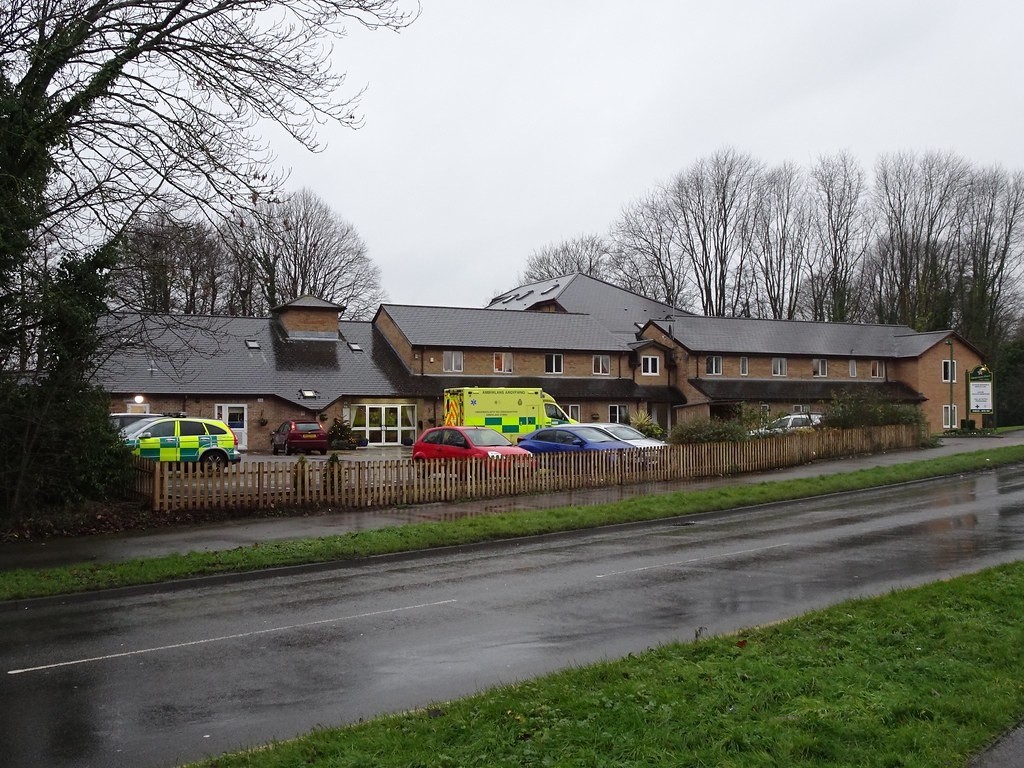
[259,418,268,426]
[428,418,436,424]
[591,413,600,420]
[320,414,328,422]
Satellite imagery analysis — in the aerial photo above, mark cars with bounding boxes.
[270,420,329,457]
[412,426,538,479]
[554,423,669,469]
[109,412,163,432]
[516,426,644,473]
[751,415,821,436]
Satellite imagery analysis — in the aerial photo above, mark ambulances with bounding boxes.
[119,411,241,477]
[443,387,581,445]
